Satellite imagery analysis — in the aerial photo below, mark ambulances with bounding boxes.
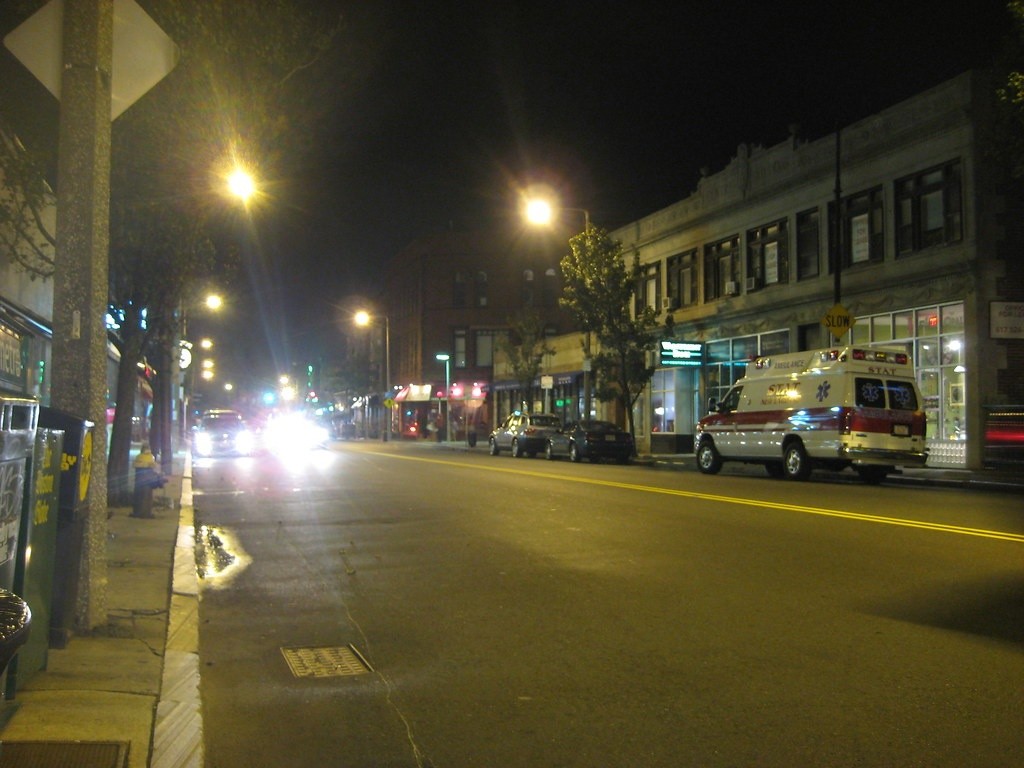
[694,344,930,485]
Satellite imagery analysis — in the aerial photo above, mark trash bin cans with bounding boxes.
[0,385,97,683]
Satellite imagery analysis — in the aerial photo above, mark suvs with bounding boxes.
[488,410,563,458]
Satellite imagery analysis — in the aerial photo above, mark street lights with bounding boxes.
[183,296,221,337]
[526,201,592,420]
[436,354,451,441]
[356,312,392,441]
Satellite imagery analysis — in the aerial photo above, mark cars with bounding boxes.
[544,420,634,463]
[195,410,253,458]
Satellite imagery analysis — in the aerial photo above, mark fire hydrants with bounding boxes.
[132,446,169,519]
